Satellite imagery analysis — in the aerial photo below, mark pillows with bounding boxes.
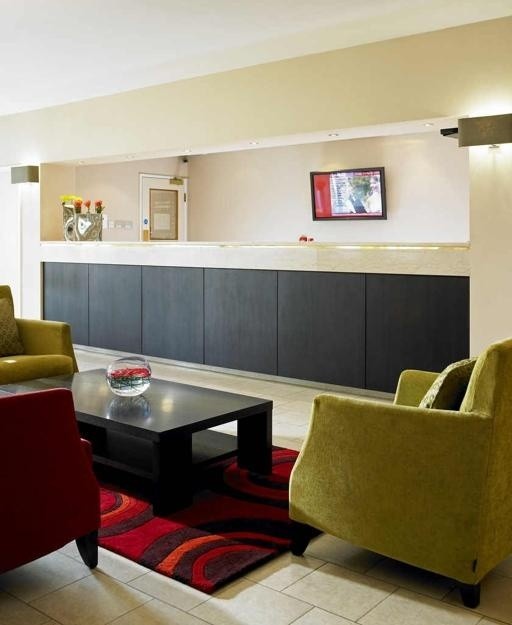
[416,355,475,411]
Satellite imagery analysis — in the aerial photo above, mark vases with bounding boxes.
[64,212,100,241]
[106,355,152,397]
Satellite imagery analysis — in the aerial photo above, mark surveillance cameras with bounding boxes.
[181,155,188,165]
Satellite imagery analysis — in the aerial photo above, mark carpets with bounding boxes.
[96,448,298,595]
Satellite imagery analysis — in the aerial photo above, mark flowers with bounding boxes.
[61,194,103,214]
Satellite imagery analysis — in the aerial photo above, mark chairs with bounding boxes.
[287,338,512,609]
[1,282,98,584]
